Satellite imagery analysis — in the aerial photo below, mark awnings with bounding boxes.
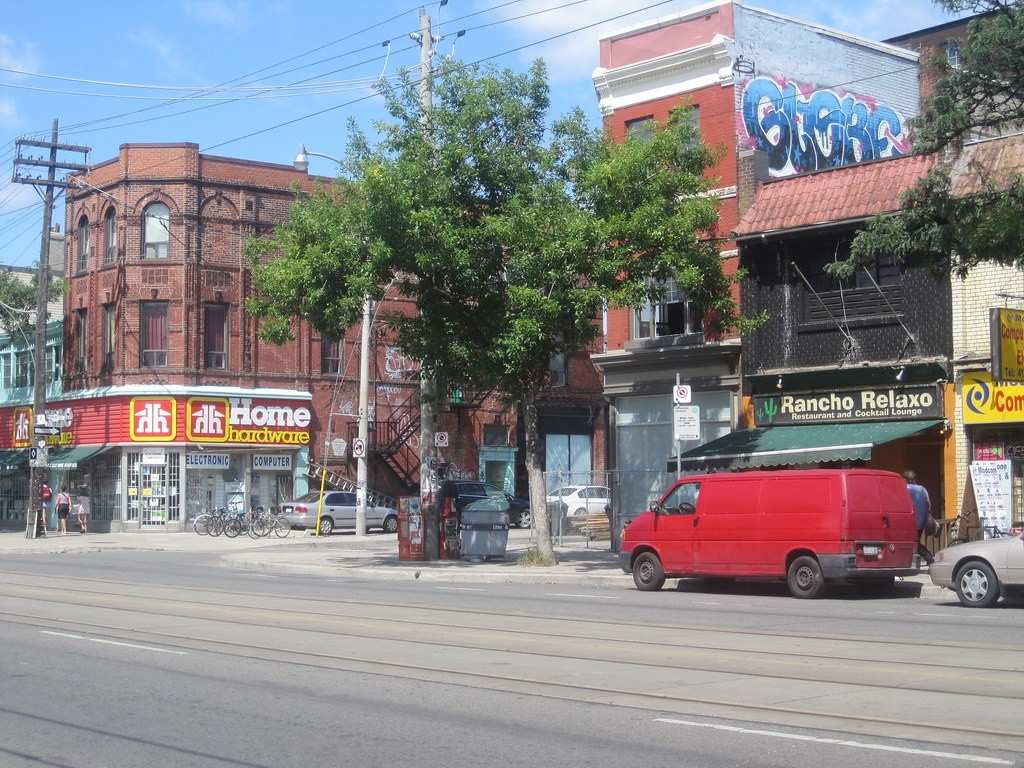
[47,446,114,471]
[667,420,944,473]
[0,451,29,470]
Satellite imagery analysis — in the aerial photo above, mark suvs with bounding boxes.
[438,479,531,529]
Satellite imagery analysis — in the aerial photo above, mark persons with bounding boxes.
[54,484,72,534]
[903,470,935,566]
[76,488,91,533]
[42,476,52,534]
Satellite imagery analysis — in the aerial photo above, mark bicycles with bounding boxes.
[194,501,292,540]
[946,507,1015,548]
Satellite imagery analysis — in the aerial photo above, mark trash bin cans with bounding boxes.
[460,497,510,563]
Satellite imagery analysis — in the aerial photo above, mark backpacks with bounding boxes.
[43,486,50,500]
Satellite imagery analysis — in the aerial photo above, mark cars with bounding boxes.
[294,146,442,566]
[278,490,398,536]
[929,528,1024,609]
[546,485,612,517]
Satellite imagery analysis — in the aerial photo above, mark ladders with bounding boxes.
[302,458,399,510]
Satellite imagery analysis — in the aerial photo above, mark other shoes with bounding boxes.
[81,529,86,533]
[927,555,935,565]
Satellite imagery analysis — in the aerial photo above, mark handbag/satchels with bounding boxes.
[925,510,935,535]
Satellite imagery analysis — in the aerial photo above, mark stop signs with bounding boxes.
[352,438,366,459]
[435,432,448,448]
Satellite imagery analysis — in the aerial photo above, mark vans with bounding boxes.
[616,468,921,599]
